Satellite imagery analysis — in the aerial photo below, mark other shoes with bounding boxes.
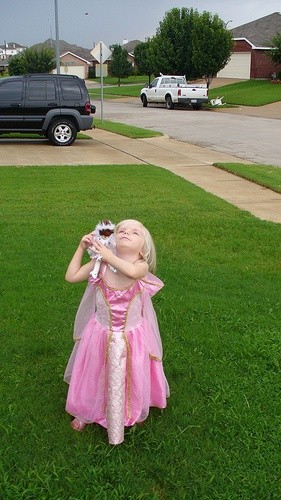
[70,417,85,431]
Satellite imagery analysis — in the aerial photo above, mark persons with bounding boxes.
[63,220,171,446]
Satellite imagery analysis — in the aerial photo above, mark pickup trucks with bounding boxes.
[140,75,209,110]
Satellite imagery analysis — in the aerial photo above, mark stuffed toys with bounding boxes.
[86,219,118,279]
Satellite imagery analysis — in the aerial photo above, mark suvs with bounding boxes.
[0,73,96,145]
